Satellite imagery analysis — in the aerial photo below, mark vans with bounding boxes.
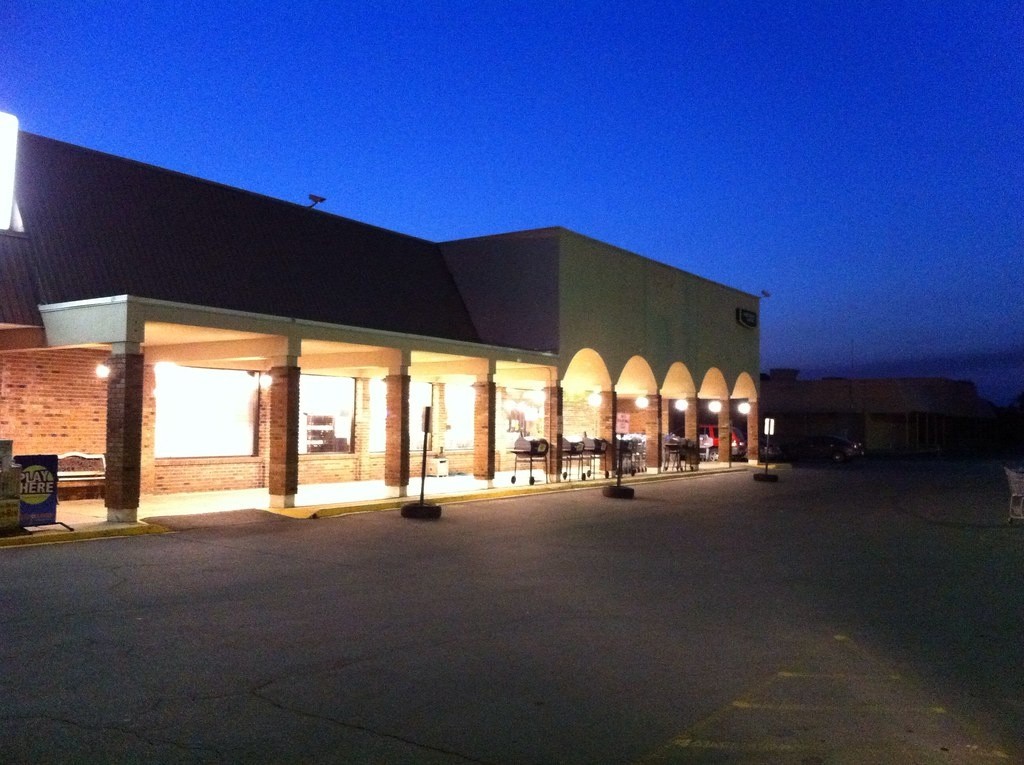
[670,424,748,464]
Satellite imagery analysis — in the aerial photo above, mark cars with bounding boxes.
[778,434,862,463]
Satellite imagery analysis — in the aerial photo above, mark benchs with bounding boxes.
[58,452,107,504]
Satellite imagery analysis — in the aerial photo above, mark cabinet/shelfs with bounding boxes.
[306,414,335,453]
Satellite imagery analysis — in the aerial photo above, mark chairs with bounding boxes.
[1004,466,1024,523]
